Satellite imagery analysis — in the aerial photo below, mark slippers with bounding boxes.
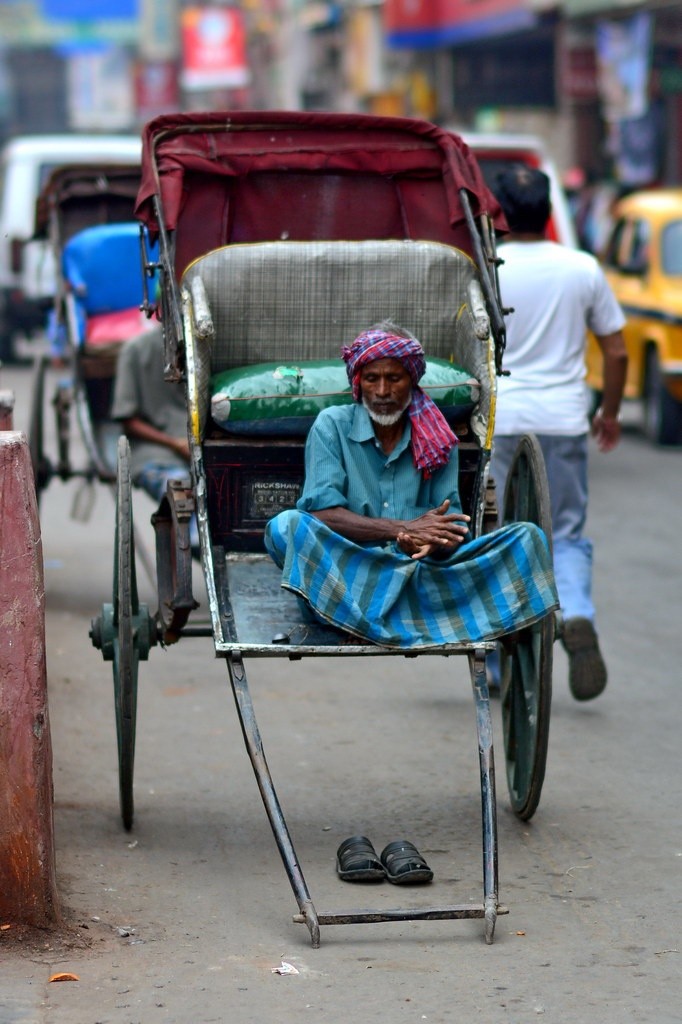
[381,840,435,884]
[337,836,387,880]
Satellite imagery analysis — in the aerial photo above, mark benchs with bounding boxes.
[61,224,167,381]
[174,239,500,465]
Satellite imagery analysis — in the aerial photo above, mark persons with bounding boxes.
[111,324,201,564]
[486,161,630,701]
[263,320,561,649]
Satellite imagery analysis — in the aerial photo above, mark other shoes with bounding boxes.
[486,684,502,699]
[563,618,608,701]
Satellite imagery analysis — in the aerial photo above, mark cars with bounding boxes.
[584,187,682,449]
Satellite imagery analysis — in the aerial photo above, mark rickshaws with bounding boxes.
[27,154,165,594]
[86,112,564,953]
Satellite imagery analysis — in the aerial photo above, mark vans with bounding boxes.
[459,129,581,250]
[0,135,145,366]
